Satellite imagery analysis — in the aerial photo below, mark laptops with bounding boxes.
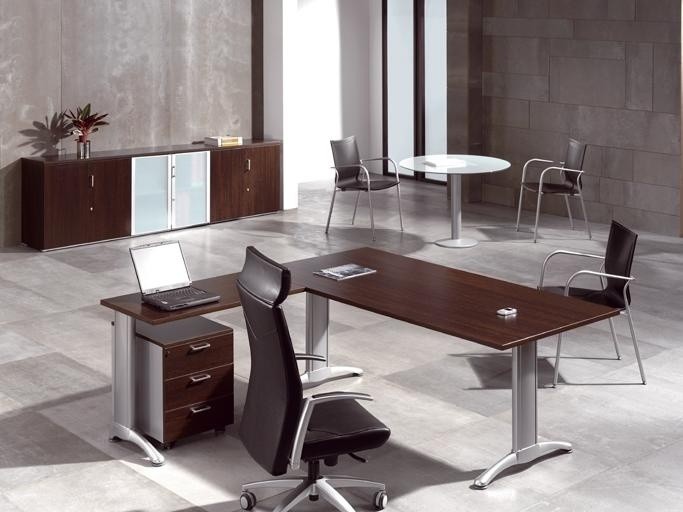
[128,240,220,312]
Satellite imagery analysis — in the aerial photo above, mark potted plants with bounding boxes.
[15,111,75,162]
[66,102,108,157]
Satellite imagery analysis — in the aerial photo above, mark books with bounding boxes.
[425,157,465,167]
[313,262,376,283]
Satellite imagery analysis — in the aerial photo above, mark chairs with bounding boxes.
[326,136,407,241]
[234,239,392,512]
[534,219,646,390]
[516,136,596,245]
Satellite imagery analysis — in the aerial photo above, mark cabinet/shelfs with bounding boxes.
[22,152,131,252]
[113,316,235,446]
[131,146,208,237]
[194,136,286,225]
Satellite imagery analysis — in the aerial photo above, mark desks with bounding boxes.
[397,150,512,246]
[96,246,620,496]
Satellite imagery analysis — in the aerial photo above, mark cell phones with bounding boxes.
[497,307,517,316]
